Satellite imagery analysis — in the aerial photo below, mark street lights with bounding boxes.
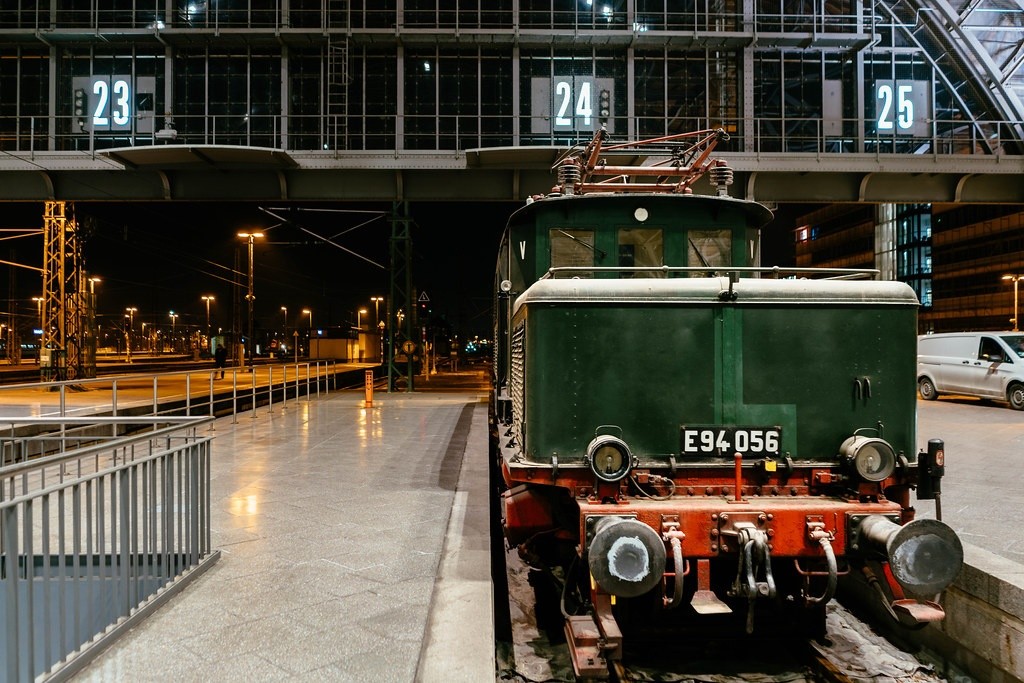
[371,297,384,325]
[87,277,102,334]
[201,295,216,360]
[236,230,265,365]
[357,309,367,332]
[302,309,312,330]
[168,314,179,354]
[281,306,288,331]
[1001,273,1024,332]
[126,307,137,357]
[31,297,45,328]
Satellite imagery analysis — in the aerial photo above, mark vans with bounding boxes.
[915,329,1024,412]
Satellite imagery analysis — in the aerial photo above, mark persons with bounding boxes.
[213,343,227,379]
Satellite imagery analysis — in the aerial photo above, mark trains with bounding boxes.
[487,122,966,683]
